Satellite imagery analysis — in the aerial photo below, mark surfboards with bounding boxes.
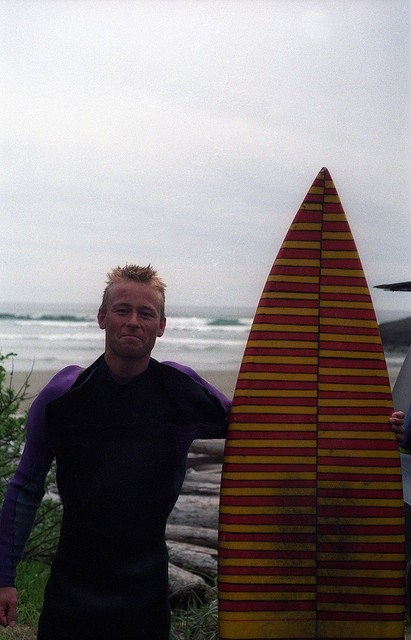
[218,168,410,640]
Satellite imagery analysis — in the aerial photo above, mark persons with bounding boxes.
[0,262,408,640]
